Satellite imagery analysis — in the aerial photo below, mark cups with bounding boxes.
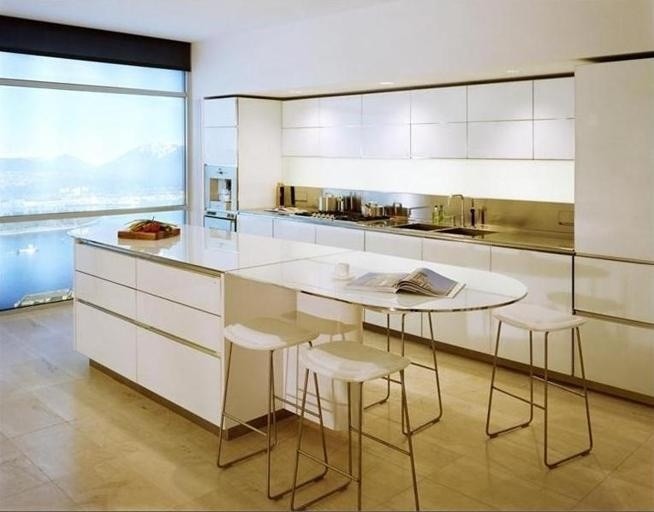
[384,206,395,217]
[396,207,407,216]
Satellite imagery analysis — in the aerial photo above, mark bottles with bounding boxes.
[432,205,439,224]
[318,194,384,218]
[439,204,446,224]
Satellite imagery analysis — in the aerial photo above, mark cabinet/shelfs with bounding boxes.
[575,54,654,410]
[280,74,574,161]
[203,95,282,168]
[74,242,296,442]
[238,212,573,383]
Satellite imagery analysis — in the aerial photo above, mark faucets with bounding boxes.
[447,193,467,228]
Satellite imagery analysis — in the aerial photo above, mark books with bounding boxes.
[343,267,466,301]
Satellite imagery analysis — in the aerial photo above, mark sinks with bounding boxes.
[433,227,501,236]
[391,223,452,232]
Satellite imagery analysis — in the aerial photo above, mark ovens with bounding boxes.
[200,162,237,215]
[202,215,236,233]
[203,228,238,255]
